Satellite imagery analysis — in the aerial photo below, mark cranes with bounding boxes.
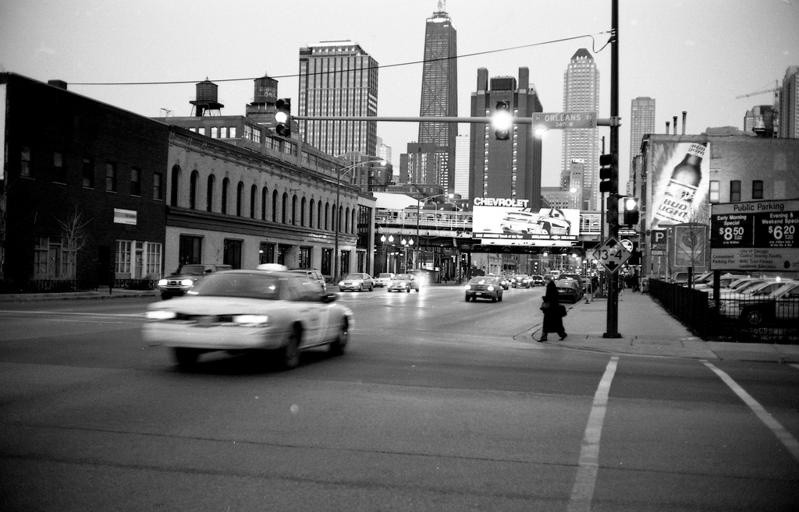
[735,79,782,138]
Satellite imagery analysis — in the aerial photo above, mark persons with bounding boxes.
[539,274,568,343]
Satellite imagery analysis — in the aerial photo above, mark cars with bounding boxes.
[555,279,581,303]
[465,274,545,302]
[671,271,799,324]
[142,262,420,369]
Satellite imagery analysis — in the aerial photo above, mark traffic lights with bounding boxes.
[495,99,511,141]
[624,198,639,225]
[275,98,292,138]
[600,154,618,193]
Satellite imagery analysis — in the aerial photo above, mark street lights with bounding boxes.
[534,128,606,298]
[380,234,394,273]
[333,159,386,285]
[400,238,414,273]
[582,258,598,301]
[415,193,454,269]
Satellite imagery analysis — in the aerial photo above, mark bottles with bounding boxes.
[651,141,707,230]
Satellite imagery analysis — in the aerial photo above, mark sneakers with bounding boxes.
[537,338,548,342]
[559,333,567,341]
